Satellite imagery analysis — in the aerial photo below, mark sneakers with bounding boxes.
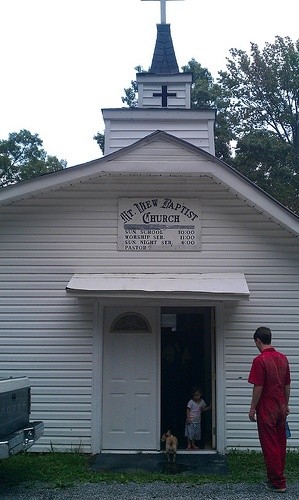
[262,481,271,486]
[266,484,287,492]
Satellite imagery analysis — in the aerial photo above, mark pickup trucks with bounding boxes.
[0,374,45,461]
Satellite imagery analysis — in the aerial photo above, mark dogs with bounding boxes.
[161,430,178,463]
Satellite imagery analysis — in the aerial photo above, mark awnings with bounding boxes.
[65,273,250,302]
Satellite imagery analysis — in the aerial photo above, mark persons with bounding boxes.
[184,388,212,450]
[248,327,291,493]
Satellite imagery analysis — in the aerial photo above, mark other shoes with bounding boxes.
[186,445,191,450]
[192,445,199,449]
